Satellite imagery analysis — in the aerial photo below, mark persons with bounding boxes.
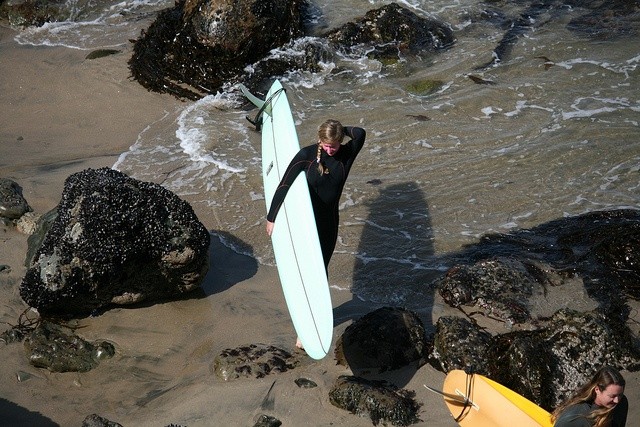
[550,364,629,426]
[267,119,366,350]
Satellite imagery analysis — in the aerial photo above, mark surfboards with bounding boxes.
[240,79,333,360]
[424,370,557,427]
